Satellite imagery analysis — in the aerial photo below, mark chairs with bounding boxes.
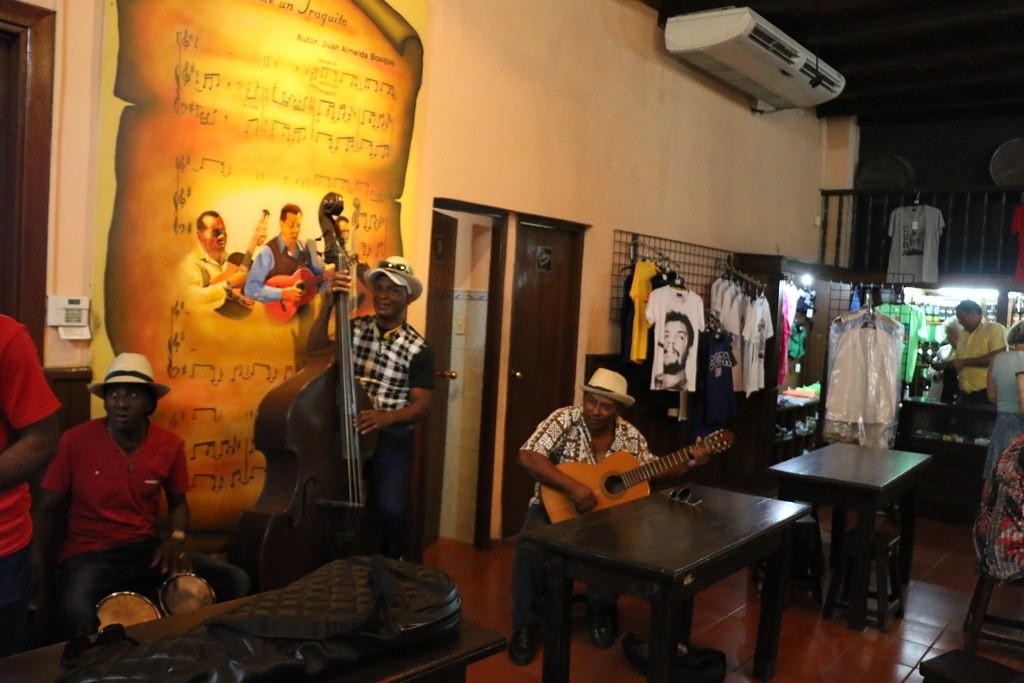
[179,539,257,605]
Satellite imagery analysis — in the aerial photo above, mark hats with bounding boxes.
[580,367,637,409]
[364,255,423,302]
[86,353,171,401]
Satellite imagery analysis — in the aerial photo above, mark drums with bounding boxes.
[158,572,217,617]
[93,591,162,634]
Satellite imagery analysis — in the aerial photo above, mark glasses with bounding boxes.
[379,260,410,276]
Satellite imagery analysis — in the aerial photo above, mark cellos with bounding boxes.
[229,187,391,595]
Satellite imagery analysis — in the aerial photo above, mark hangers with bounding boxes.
[854,282,927,332]
[715,259,765,301]
[901,187,933,218]
[781,267,815,290]
[641,236,684,285]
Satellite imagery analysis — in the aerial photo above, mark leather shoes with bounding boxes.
[508,621,536,665]
[586,595,621,647]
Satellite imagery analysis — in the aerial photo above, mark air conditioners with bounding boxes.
[664,7,846,113]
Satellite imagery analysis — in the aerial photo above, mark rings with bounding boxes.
[332,287,336,292]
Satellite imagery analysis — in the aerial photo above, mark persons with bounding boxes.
[926,298,1024,587]
[507,366,710,666]
[30,352,253,646]
[308,255,434,567]
[1,313,63,656]
[653,310,695,392]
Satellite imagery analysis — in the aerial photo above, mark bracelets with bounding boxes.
[170,531,187,540]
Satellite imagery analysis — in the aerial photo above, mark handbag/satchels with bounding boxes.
[971,431,1024,583]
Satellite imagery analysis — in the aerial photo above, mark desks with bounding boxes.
[0,557,510,683]
[769,443,933,633]
[511,482,814,683]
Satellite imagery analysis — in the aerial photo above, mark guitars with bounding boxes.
[537,428,739,526]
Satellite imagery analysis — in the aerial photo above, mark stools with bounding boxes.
[961,574,1024,671]
[920,650,1024,683]
[826,527,906,631]
